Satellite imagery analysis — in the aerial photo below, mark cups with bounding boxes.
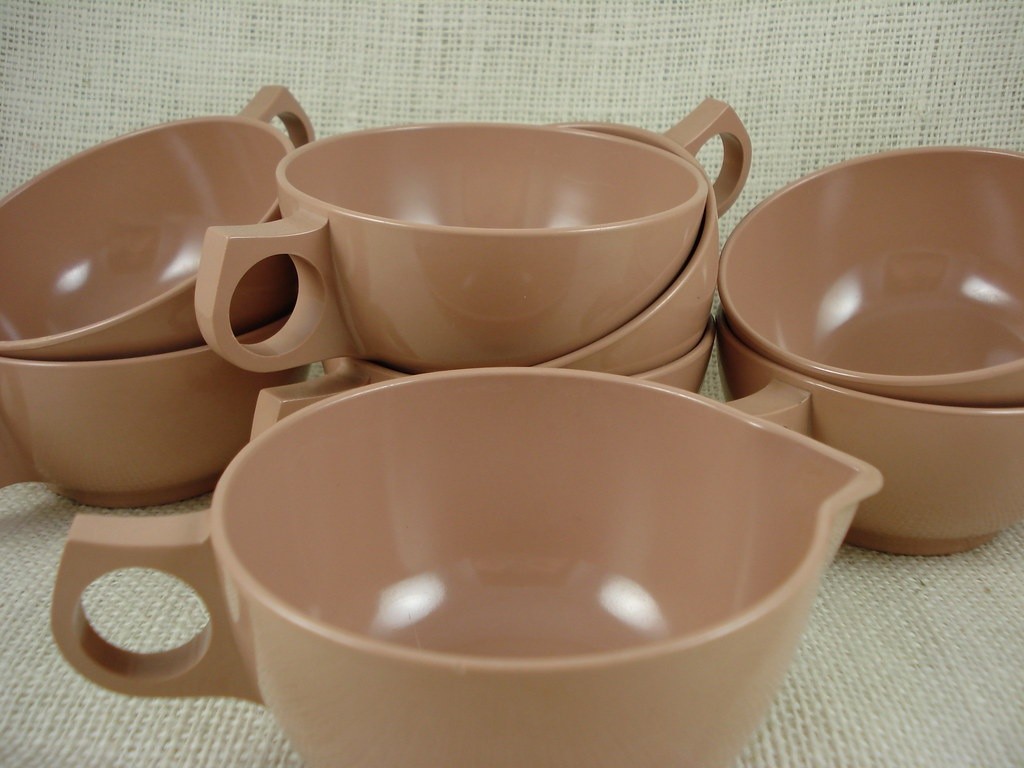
[0,85,1024,768]
